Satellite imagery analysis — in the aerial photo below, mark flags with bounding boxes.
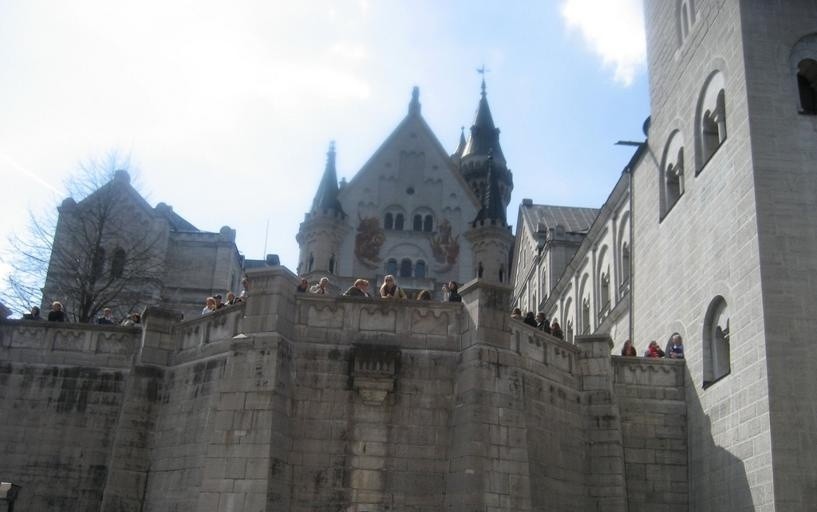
[476,69,482,74]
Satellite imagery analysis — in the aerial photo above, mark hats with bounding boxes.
[213,294,222,299]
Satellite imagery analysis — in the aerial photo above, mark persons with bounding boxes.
[132,313,140,323]
[20,307,45,321]
[416,289,432,300]
[309,276,328,294]
[536,312,548,331]
[441,284,450,301]
[551,322,563,339]
[523,312,537,327]
[123,314,135,327]
[95,307,114,325]
[48,301,70,321]
[511,308,525,321]
[379,275,408,301]
[361,280,371,298]
[622,340,636,356]
[298,278,308,292]
[201,277,248,315]
[448,280,461,302]
[644,341,665,358]
[342,279,364,297]
[669,334,684,358]
